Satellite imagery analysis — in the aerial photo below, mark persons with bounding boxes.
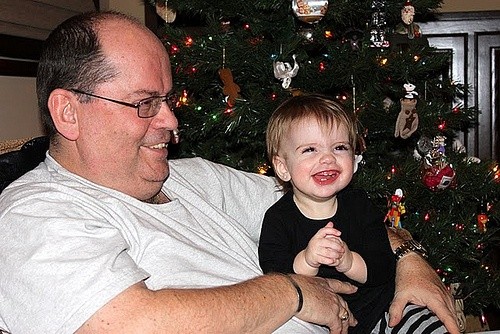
[256,93,395,334]
[2,10,465,334]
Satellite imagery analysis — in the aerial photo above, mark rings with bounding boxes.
[341,308,350,324]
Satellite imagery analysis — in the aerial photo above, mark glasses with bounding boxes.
[70,88,178,118]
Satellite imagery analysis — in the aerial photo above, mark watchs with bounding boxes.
[392,238,429,262]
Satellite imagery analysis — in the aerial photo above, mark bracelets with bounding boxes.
[282,270,304,316]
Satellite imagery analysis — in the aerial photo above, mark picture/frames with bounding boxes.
[1,1,106,81]
